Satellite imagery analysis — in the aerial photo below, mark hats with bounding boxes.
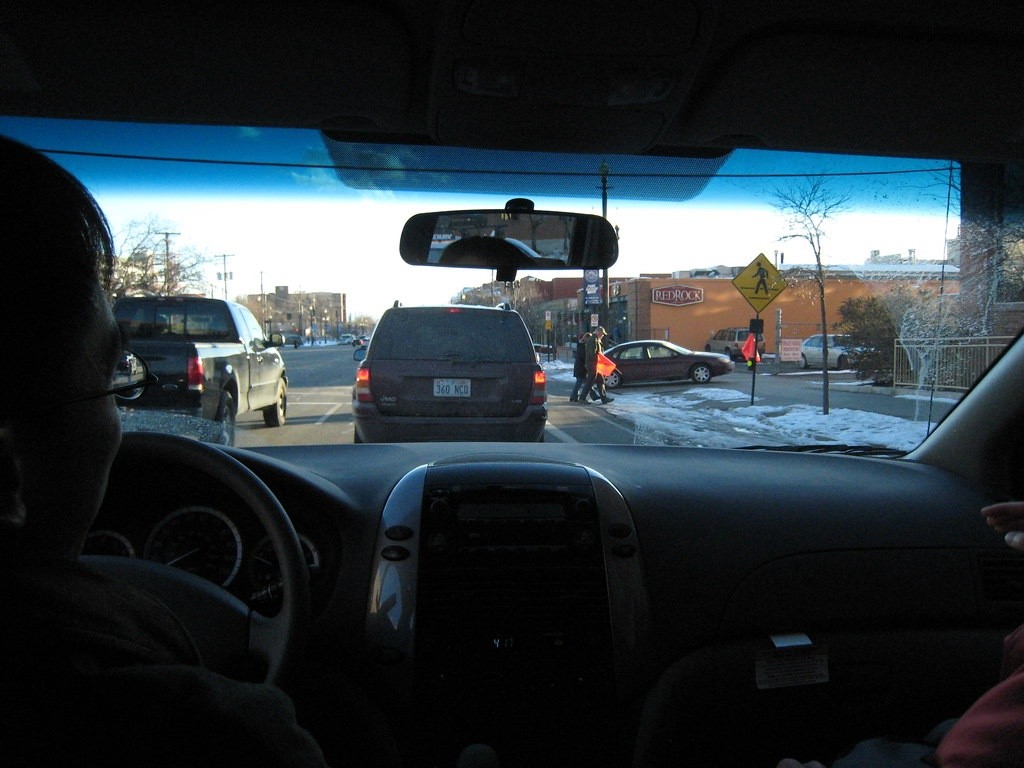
[595,326,607,336]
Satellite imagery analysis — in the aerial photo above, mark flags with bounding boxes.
[597,351,615,376]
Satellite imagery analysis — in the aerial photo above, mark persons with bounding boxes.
[777,503,1024,768]
[1,135,327,768]
[570,326,615,406]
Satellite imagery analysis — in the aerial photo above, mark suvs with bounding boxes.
[703,325,765,361]
[350,299,548,443]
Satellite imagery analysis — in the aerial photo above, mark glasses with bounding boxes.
[49,349,159,409]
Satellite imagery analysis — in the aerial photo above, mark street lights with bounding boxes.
[593,158,615,352]
[154,232,181,296]
[214,254,235,300]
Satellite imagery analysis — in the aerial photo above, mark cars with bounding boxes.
[594,339,736,390]
[337,332,370,346]
[796,333,882,371]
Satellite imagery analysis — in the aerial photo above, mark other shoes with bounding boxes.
[578,397,591,406]
[570,396,578,402]
[601,396,614,405]
[591,394,601,401]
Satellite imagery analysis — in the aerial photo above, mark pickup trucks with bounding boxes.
[111,293,289,447]
